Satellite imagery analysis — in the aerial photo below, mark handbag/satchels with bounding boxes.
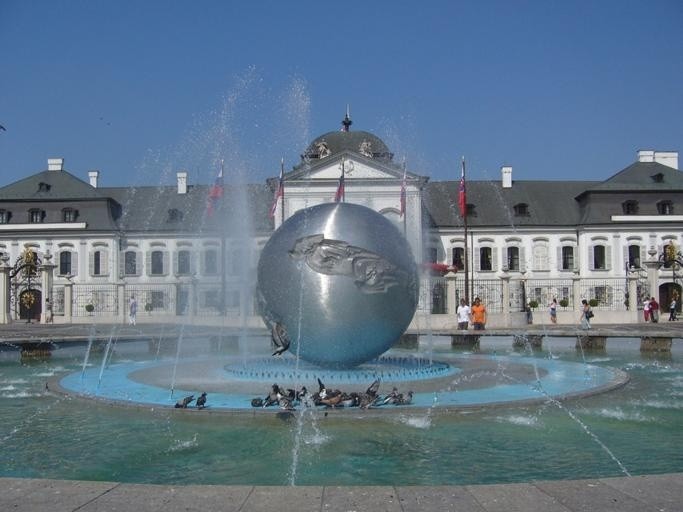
[586,311,594,319]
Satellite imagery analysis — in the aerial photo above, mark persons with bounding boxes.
[456,299,471,330]
[580,299,591,330]
[644,296,676,323]
[129,295,138,326]
[471,297,487,330]
[550,298,557,324]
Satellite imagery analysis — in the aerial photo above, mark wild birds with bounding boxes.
[271,322,290,356]
[251,377,416,412]
[175,395,196,409]
[195,392,207,409]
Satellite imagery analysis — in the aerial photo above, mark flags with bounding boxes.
[400,161,406,217]
[336,164,344,201]
[460,162,466,216]
[206,169,223,216]
[268,163,283,218]
